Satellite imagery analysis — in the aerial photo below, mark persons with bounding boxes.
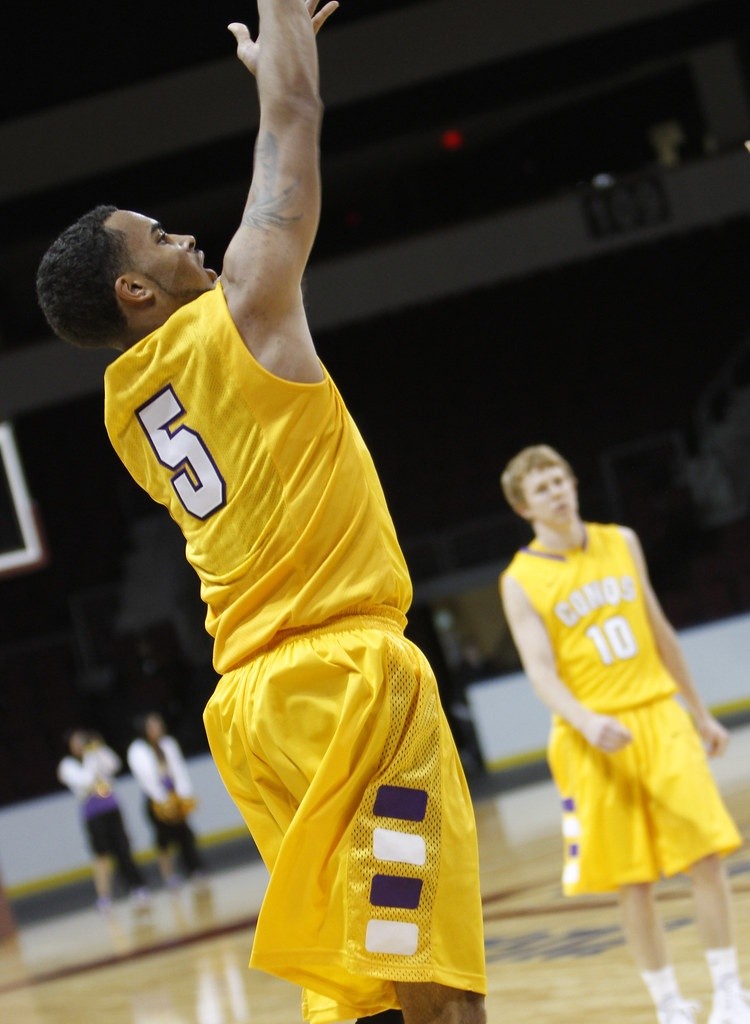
[126,713,201,889]
[498,445,749,1024]
[55,729,147,908]
[34,1,490,1024]
[451,638,496,718]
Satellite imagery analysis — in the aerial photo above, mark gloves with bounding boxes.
[151,794,180,823]
[177,796,196,816]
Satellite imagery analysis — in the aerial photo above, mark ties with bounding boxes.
[152,742,167,767]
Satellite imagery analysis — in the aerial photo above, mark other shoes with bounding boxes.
[656,999,701,1024]
[708,988,750,1024]
